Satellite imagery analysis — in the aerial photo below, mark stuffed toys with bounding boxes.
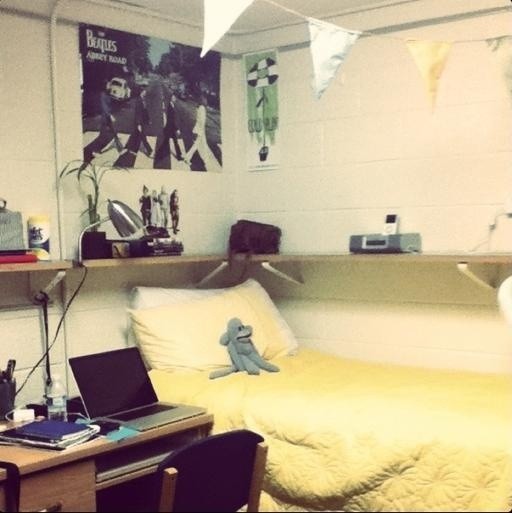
[209,318,280,380]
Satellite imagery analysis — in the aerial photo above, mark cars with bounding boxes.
[106,77,131,101]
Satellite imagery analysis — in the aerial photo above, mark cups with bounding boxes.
[0,383,16,421]
[27,216,50,260]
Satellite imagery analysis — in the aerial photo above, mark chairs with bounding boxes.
[147,430,269,512]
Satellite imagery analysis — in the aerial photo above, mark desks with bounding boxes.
[0,410,214,513]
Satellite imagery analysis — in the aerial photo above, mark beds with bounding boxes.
[148,350,511,512]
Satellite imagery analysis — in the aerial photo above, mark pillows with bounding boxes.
[124,279,299,371]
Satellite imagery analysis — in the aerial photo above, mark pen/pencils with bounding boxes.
[6,360,13,382]
[13,360,18,375]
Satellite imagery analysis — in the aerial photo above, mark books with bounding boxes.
[0,418,101,451]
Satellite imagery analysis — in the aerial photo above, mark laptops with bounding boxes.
[68,346,207,431]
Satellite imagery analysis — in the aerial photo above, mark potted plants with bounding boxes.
[60,155,129,259]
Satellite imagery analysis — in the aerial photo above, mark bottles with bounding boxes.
[46,376,67,422]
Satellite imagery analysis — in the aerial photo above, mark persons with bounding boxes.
[84,79,123,162]
[131,87,152,156]
[140,185,180,234]
[184,93,212,164]
[153,94,184,160]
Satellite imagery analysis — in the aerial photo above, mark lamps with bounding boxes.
[74,200,145,264]
